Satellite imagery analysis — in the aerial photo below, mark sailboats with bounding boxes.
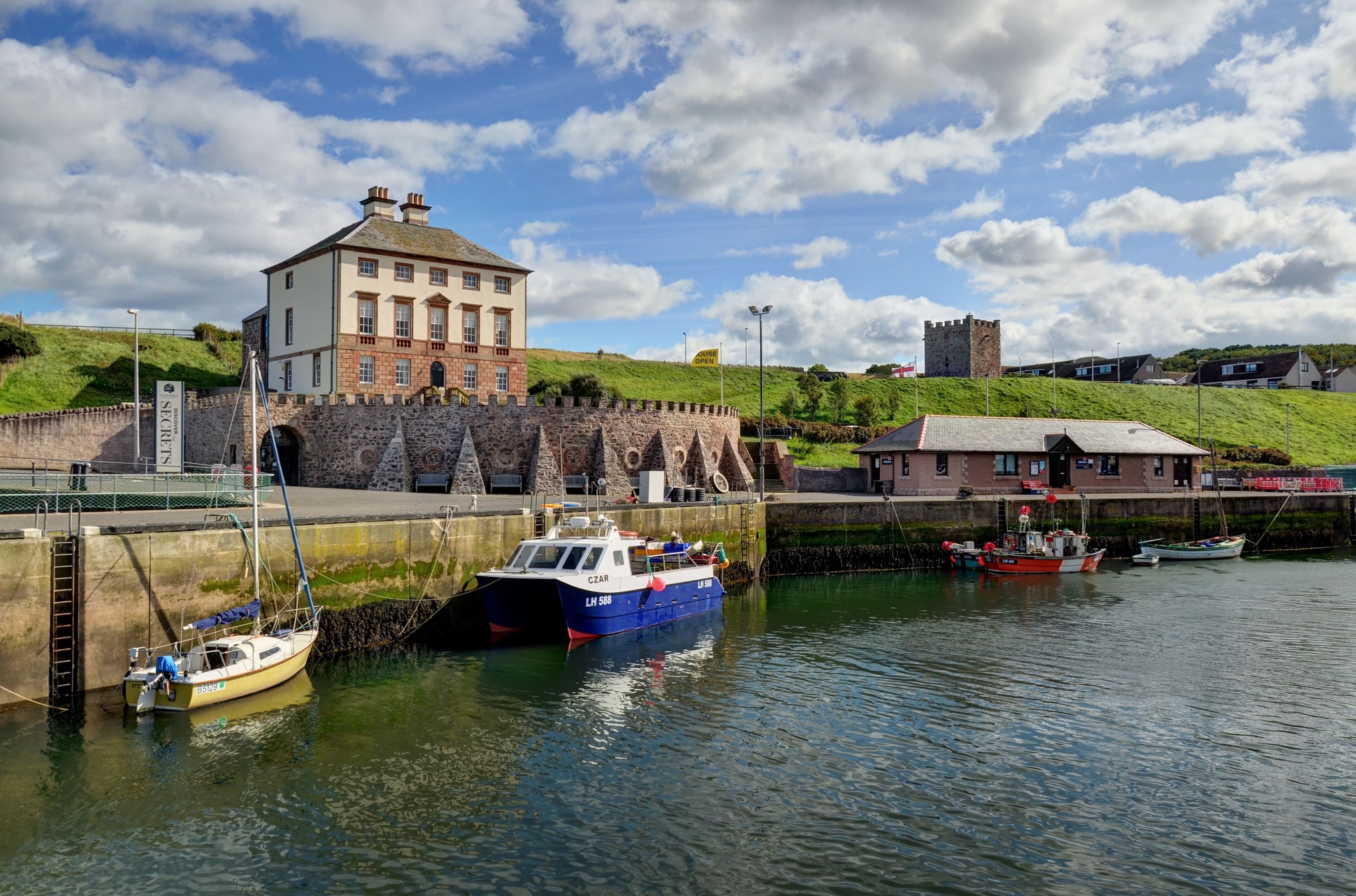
[122,351,323,715]
[1140,443,1247,559]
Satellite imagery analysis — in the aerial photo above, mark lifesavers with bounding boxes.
[1005,537,1016,551]
[1057,530,1075,536]
[618,530,638,540]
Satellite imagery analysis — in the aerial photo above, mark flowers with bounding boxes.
[1070,484,1077,487]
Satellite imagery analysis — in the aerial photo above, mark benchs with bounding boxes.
[628,477,640,489]
[1216,478,1243,491]
[563,475,593,494]
[490,474,522,494]
[416,473,449,493]
[1021,480,1050,494]
[682,475,692,485]
[1242,477,1262,491]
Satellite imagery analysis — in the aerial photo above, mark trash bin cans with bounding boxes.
[244,466,258,490]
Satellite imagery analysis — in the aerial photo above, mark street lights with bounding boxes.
[683,332,686,362]
[984,372,989,416]
[720,342,723,406]
[1048,369,1056,413]
[745,328,748,369]
[1018,357,1021,375]
[1281,403,1289,454]
[1196,359,1208,448]
[914,356,918,419]
[1092,349,1095,381]
[749,305,774,502]
[128,308,140,473]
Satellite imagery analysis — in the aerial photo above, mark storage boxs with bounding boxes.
[541,502,581,508]
[628,535,719,575]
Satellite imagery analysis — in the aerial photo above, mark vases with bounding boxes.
[1073,487,1075,491]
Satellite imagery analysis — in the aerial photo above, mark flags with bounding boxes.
[691,348,719,367]
[891,360,915,378]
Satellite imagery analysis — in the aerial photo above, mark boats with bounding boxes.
[945,527,1109,571]
[1133,551,1160,566]
[470,513,730,643]
